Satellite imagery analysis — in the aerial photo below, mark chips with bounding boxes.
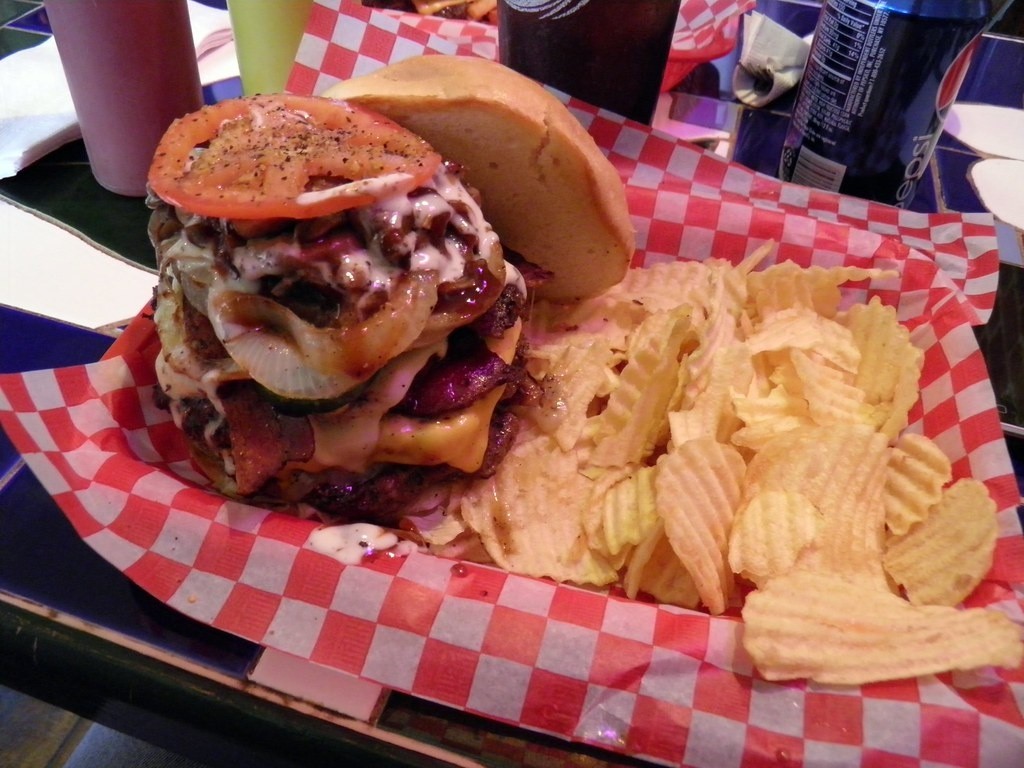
[400,232,1022,682]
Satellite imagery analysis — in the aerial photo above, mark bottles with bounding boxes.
[41,0,205,195]
[496,0,680,126]
[225,0,314,97]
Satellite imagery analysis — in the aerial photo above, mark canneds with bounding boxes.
[779,0,988,211]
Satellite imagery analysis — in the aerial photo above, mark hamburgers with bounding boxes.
[143,54,636,540]
